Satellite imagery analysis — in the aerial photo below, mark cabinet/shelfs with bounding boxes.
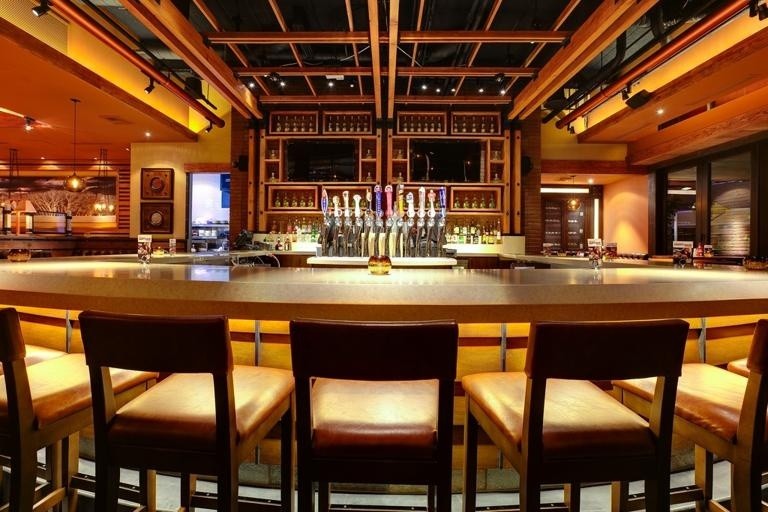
[258,110,510,236]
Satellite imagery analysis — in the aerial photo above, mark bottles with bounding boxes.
[269,150,278,159]
[260,216,321,251]
[364,149,373,159]
[446,217,503,244]
[492,148,501,160]
[271,114,316,132]
[396,149,404,159]
[492,173,501,183]
[365,172,372,181]
[454,192,496,208]
[328,115,370,132]
[268,172,278,182]
[321,182,447,258]
[274,192,314,207]
[453,115,496,133]
[396,172,404,182]
[401,115,442,132]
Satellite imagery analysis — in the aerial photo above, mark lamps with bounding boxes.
[567,176,581,211]
[63,102,85,192]
